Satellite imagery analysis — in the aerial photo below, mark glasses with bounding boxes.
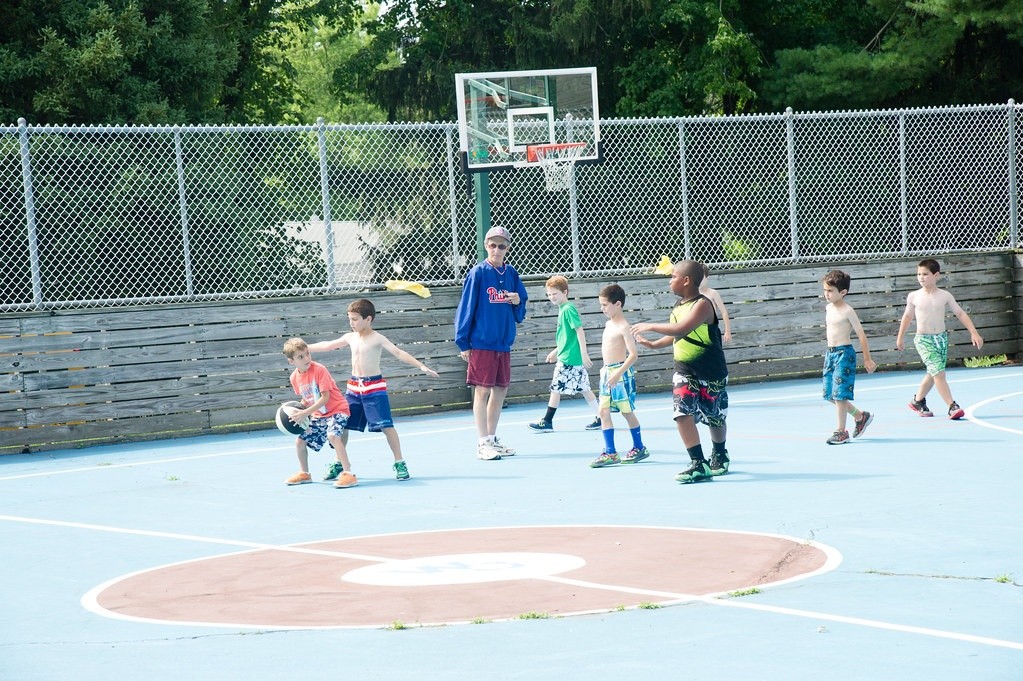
[486,243,508,250]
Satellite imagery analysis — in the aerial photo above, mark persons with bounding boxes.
[454,226,529,460]
[822,270,877,445]
[307,299,439,481]
[630,260,730,483]
[283,338,358,488]
[896,259,984,419]
[698,264,732,342]
[528,276,602,433]
[588,284,650,468]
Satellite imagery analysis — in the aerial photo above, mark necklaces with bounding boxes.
[486,259,506,275]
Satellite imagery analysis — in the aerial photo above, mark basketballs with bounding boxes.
[276,401,310,435]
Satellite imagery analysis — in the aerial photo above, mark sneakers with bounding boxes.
[332,471,360,488]
[620,445,650,464]
[392,460,411,481]
[322,461,352,481]
[948,400,965,420]
[585,416,602,430]
[285,471,313,485]
[476,439,502,460]
[825,429,851,445]
[708,448,730,477]
[491,436,517,457]
[588,448,621,468]
[908,394,933,417]
[674,459,714,483]
[527,417,554,432]
[852,411,874,439]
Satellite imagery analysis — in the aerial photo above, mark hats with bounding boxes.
[484,225,511,243]
